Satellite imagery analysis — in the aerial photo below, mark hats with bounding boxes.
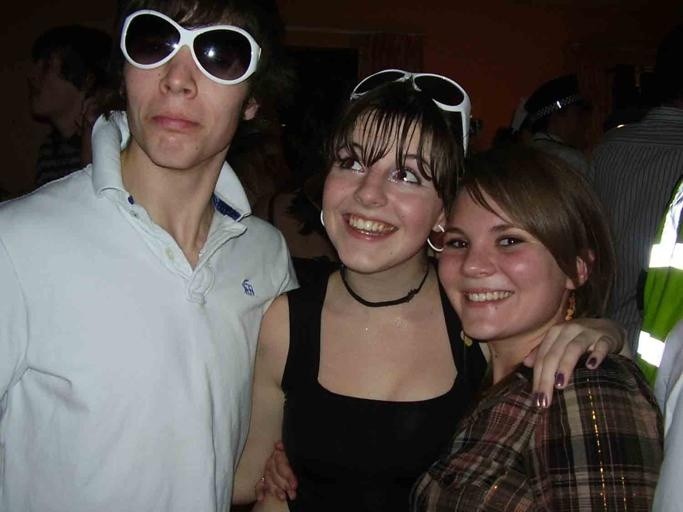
[524,74,584,127]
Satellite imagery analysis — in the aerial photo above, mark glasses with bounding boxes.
[349,68,473,160]
[120,9,263,85]
